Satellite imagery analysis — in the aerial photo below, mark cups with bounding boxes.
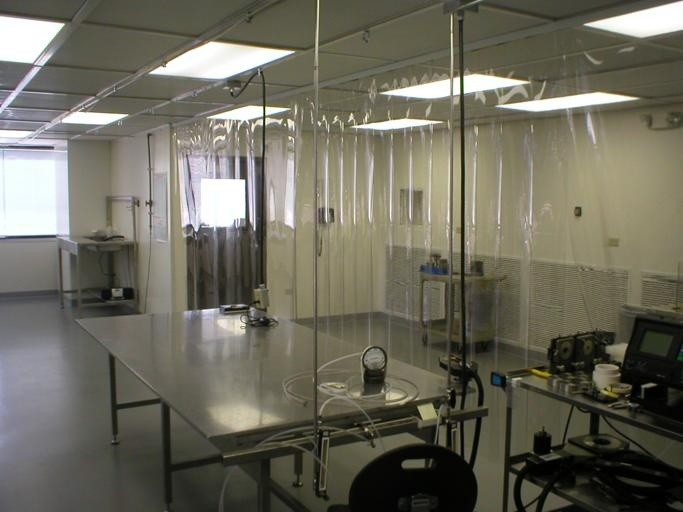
[591,363,622,392]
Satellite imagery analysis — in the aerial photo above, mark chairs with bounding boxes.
[329,443,478,511]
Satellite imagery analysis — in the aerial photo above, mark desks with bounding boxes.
[56,236,138,315]
[73,304,475,512]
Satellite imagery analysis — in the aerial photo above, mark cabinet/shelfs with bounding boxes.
[491,364,683,512]
